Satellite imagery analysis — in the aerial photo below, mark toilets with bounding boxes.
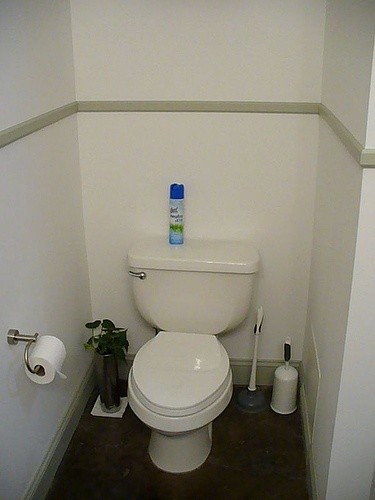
[126,236,261,474]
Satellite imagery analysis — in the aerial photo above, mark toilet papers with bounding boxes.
[6,329,68,385]
